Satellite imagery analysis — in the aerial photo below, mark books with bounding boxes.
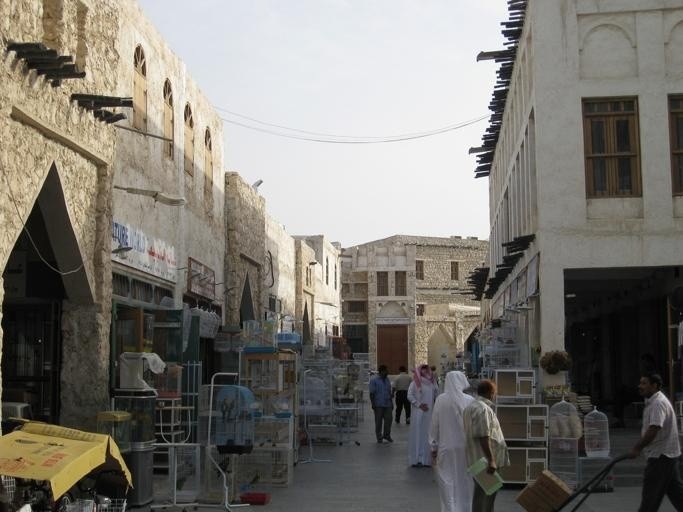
[467,456,504,497]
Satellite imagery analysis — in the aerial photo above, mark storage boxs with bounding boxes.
[514,465,572,512]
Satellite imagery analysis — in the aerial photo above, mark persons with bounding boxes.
[407,363,436,469]
[462,378,512,512]
[429,364,440,403]
[391,365,411,425]
[425,369,479,512]
[369,364,394,444]
[625,370,683,512]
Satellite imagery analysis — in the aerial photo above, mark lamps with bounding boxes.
[113,184,187,209]
[109,228,136,261]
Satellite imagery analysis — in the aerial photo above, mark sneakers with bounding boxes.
[394,417,410,424]
[376,434,393,444]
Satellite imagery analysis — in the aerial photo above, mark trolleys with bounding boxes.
[549,450,637,512]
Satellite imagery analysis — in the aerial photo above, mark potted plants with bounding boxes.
[539,350,569,376]
